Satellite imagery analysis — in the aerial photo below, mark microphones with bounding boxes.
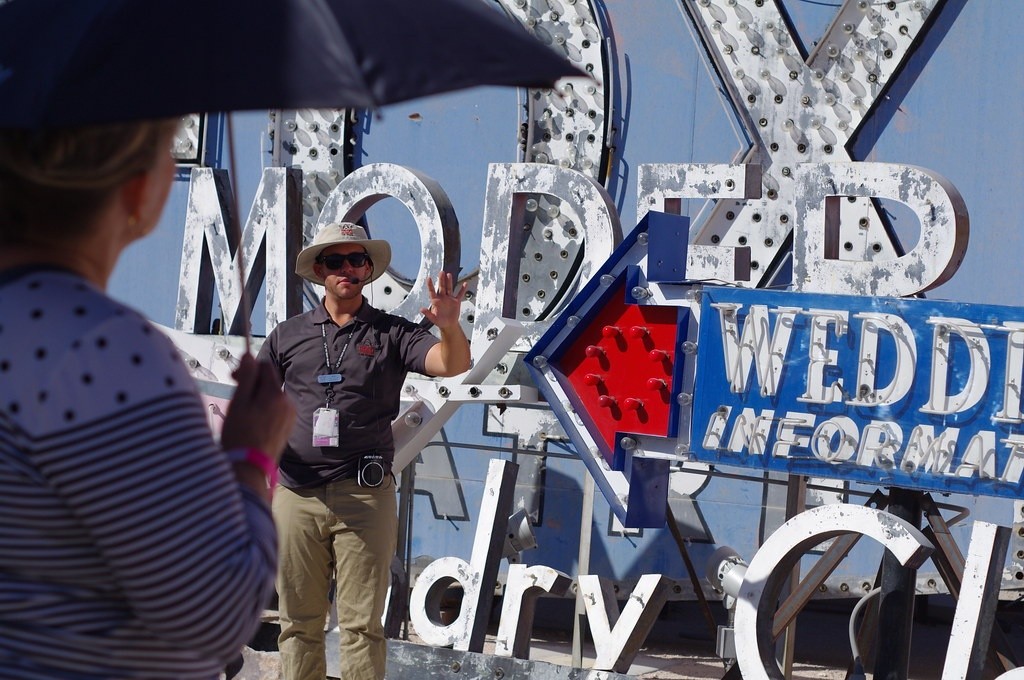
[350,257,374,285]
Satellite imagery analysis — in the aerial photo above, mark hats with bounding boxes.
[295,222,391,286]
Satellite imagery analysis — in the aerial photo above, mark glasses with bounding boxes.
[319,251,368,270]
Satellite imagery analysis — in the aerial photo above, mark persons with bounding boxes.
[0,0,298,680]
[251,223,477,680]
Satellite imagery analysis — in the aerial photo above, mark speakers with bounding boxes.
[357,455,385,489]
